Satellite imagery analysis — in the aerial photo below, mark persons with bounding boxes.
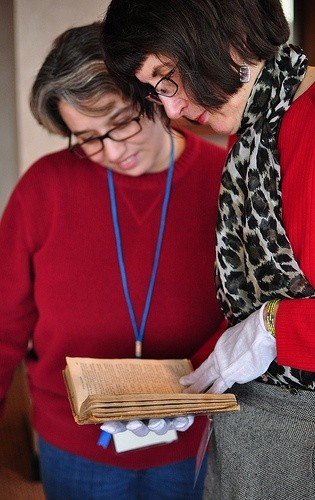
[0,20,231,500]
[95,0,314,500]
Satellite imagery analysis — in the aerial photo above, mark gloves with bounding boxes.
[101,416,194,435]
[179,295,278,395]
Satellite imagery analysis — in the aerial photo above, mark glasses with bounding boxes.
[143,60,178,105]
[68,107,144,159]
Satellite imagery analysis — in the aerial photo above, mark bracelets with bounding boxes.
[265,297,281,339]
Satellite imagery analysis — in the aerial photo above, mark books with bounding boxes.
[52,354,243,423]
